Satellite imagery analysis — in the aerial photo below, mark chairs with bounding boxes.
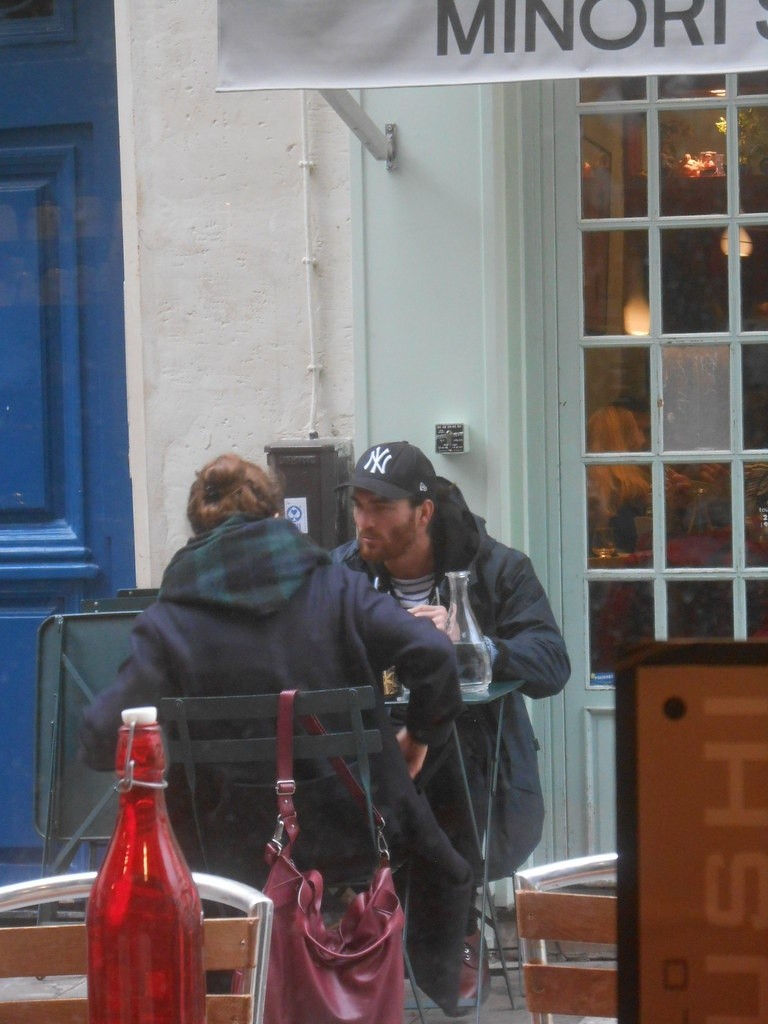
[31,589,177,921]
[161,685,425,1024]
[513,854,619,1024]
[0,868,273,1024]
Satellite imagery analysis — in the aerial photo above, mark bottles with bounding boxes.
[442,570,492,696]
[86,706,207,1024]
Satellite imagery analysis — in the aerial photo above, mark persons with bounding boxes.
[76,453,462,995]
[588,370,768,550]
[325,440,573,1017]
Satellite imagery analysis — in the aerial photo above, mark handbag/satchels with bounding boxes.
[233,688,405,1024]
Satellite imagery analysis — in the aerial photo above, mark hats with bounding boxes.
[334,440,436,503]
[612,395,651,411]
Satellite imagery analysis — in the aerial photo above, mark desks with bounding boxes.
[396,679,526,1024]
